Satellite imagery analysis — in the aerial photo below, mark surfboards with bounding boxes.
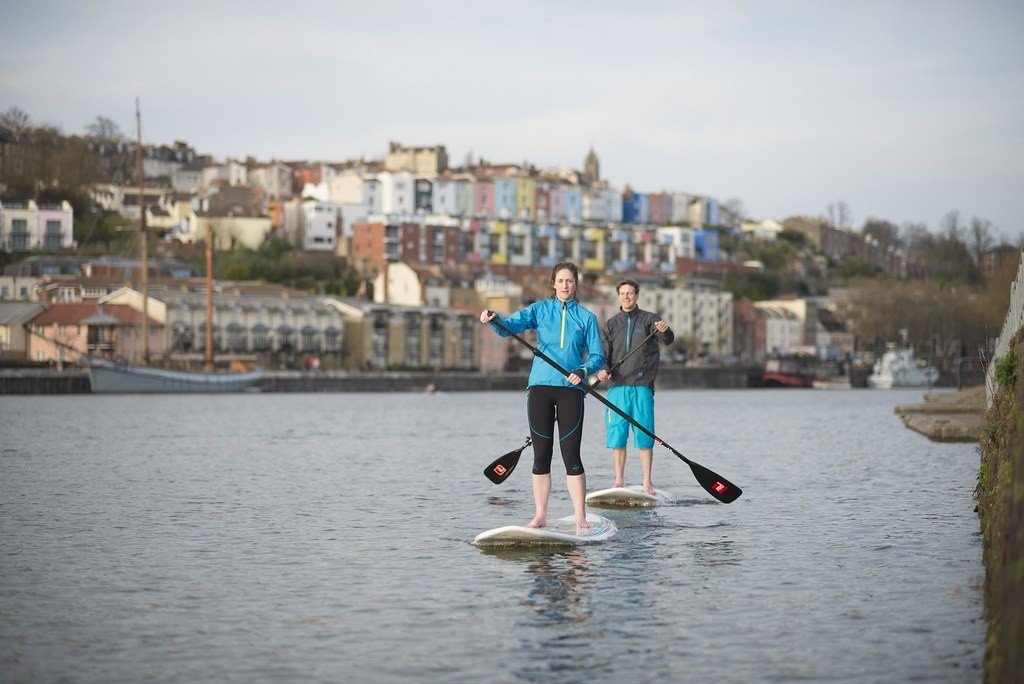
[585,484,669,502]
[474,512,618,544]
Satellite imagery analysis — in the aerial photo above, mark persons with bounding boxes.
[598,277,674,494]
[481,262,606,529]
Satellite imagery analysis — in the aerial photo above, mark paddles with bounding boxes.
[483,327,658,485]
[487,311,743,504]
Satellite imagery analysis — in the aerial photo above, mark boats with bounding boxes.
[866,328,940,390]
[87,360,269,395]
[762,346,852,389]
